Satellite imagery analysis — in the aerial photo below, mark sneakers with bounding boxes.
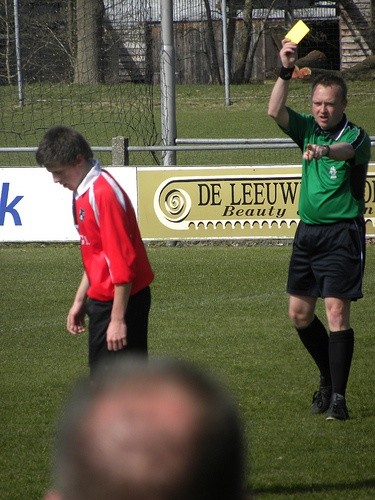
[326,393,348,420]
[311,387,331,414]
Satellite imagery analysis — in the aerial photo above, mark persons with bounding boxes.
[35,126,156,378]
[266,38,370,420]
[47,354,245,500]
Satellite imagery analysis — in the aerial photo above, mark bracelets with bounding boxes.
[320,144,330,156]
